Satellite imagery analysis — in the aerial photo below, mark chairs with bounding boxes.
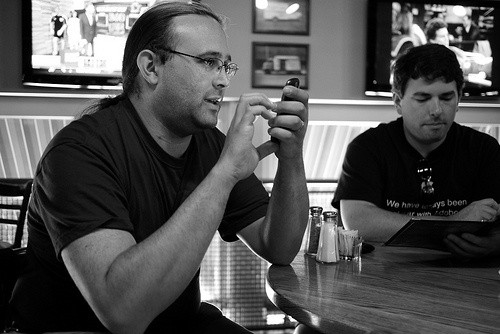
[0,177,34,251]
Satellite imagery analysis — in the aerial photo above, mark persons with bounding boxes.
[395,10,480,59]
[79,4,96,57]
[22,2,309,334]
[331,43,500,255]
[50,7,68,56]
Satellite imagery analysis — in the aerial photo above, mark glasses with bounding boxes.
[160,46,240,79]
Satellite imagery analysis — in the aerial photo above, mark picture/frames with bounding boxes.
[251,0,311,35]
[251,41,311,89]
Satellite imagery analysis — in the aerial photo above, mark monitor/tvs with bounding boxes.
[365,0,500,100]
[22,0,166,89]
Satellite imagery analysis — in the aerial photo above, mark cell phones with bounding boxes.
[270,78,300,142]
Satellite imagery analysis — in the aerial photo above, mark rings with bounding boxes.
[481,219,484,222]
[300,121,304,128]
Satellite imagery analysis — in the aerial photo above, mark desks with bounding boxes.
[262,242,500,334]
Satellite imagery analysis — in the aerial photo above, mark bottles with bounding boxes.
[305,206,325,256]
[315,211,341,263]
